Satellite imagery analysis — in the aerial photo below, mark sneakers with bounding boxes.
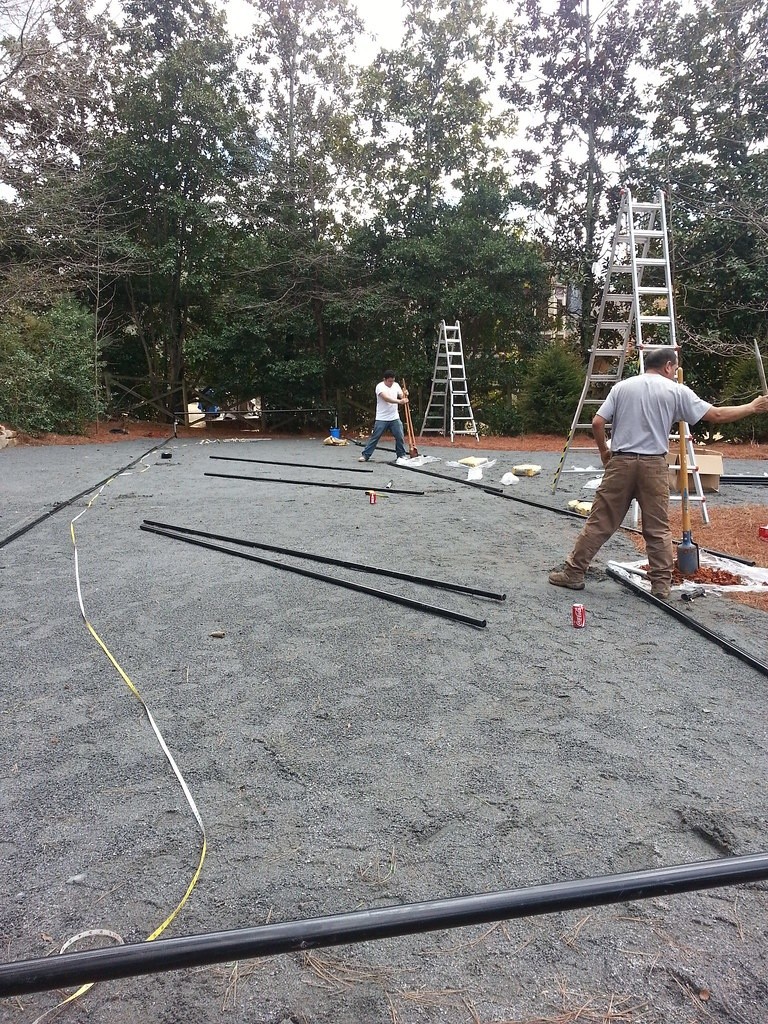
[651,588,670,598]
[549,567,586,589]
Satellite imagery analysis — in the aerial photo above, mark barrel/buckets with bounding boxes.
[330,428,341,439]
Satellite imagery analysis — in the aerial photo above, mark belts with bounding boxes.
[613,451,637,456]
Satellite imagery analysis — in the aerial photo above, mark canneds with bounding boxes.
[572,604,585,628]
[370,492,377,504]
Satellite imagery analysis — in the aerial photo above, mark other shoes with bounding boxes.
[401,456,408,460]
[358,456,367,462]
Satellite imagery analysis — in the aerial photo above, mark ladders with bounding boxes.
[552,187,710,529]
[419,319,480,442]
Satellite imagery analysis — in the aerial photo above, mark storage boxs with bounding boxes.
[666,449,724,492]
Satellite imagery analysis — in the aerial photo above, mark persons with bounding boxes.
[548,348,768,597]
[358,370,409,462]
